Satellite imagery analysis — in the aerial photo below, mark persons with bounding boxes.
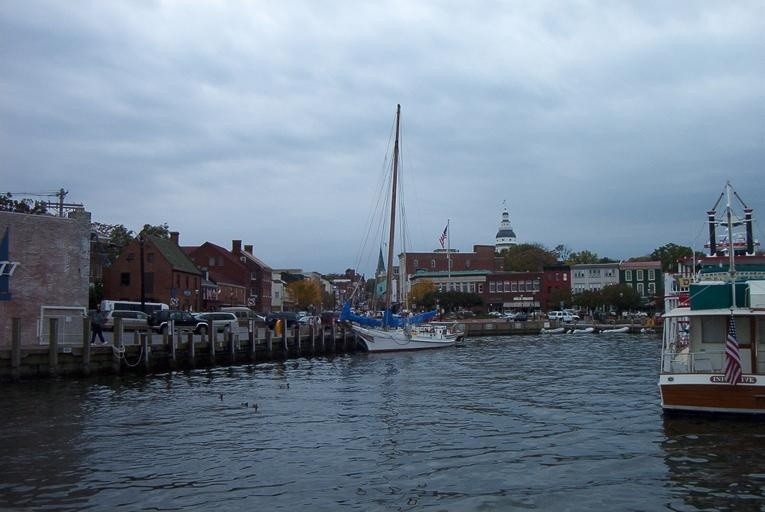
[90,309,108,345]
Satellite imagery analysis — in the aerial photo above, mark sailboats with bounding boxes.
[338,105,464,352]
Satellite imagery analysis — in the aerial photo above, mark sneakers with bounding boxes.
[90,341,108,346]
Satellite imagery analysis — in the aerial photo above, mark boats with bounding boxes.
[540,326,629,333]
[658,181,760,414]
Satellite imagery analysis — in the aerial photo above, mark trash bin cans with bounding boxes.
[264,317,277,337]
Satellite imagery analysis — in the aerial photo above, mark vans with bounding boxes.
[100,300,266,335]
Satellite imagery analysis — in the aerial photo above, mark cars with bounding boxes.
[267,311,321,328]
[488,308,647,322]
[350,301,401,318]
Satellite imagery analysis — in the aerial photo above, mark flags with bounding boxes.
[439,224,448,249]
[724,318,743,386]
